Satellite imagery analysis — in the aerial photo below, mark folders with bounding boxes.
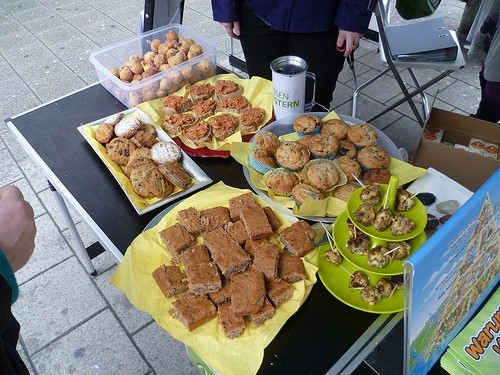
[383,17,457,61]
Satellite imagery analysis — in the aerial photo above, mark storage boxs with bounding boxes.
[409,88,500,194]
[90,22,218,109]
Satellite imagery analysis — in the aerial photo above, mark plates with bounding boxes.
[134,190,314,338]
[243,111,403,223]
[75,106,213,216]
[160,79,272,158]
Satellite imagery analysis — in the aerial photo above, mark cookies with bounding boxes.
[423,126,498,154]
[95,112,192,198]
[416,193,460,239]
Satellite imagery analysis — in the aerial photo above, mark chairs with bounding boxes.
[351,0,467,130]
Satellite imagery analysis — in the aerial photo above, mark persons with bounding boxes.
[0,184,37,375]
[472,24,500,124]
[211,0,377,111]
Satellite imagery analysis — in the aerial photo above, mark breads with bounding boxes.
[163,78,264,145]
[151,192,316,338]
[106,29,217,105]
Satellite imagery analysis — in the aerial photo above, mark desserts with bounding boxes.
[249,115,390,205]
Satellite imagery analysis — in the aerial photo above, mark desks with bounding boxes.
[5,66,403,375]
